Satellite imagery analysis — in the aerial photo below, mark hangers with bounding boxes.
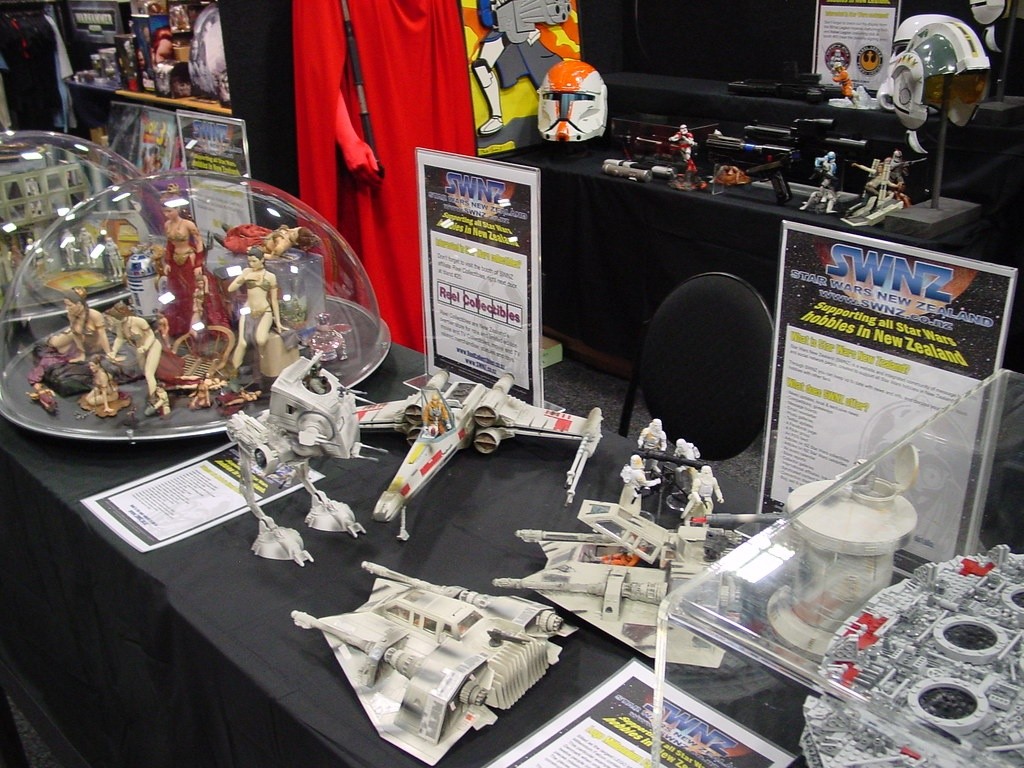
[0,0,53,21]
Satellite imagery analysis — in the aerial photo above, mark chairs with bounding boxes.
[617,271,774,462]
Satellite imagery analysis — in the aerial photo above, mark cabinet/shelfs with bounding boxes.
[53,0,299,248]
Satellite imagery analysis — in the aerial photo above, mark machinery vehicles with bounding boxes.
[290,561,1024,768]
[485,482,785,666]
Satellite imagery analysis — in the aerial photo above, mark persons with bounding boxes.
[832,62,855,104]
[60,228,123,278]
[154,28,173,63]
[669,125,708,189]
[9,238,49,279]
[845,150,912,218]
[800,151,838,213]
[43,287,172,415]
[158,194,331,407]
[620,418,724,527]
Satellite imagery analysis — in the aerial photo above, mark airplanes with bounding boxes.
[355,366,605,542]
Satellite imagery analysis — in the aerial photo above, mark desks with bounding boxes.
[0,300,928,768]
